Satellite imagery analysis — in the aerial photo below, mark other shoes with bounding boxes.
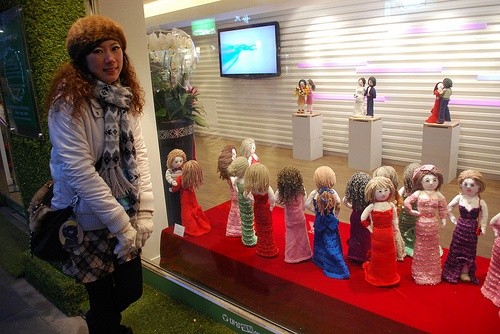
[84,310,118,334]
[110,322,132,334]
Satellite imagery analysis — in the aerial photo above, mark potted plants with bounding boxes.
[149,50,209,227]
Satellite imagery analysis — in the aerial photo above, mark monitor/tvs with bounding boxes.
[218,21,281,78]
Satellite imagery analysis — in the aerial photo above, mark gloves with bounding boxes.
[113,226,137,258]
[135,215,153,248]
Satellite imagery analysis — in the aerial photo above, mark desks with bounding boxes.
[159,200,500,334]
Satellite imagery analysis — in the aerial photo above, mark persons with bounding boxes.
[217,144,243,237]
[343,171,374,262]
[425,78,453,124]
[481,213,500,307]
[237,137,261,166]
[165,148,187,226]
[46,15,155,334]
[442,169,489,285]
[373,166,402,216]
[295,79,316,114]
[403,164,448,286]
[398,163,443,259]
[364,77,376,116]
[352,78,367,117]
[305,166,351,279]
[277,165,313,263]
[244,163,280,256]
[169,159,211,236]
[361,177,400,287]
[227,156,258,246]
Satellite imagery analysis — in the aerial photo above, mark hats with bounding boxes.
[67,16,126,60]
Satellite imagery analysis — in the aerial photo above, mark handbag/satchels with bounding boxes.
[29,178,75,270]
